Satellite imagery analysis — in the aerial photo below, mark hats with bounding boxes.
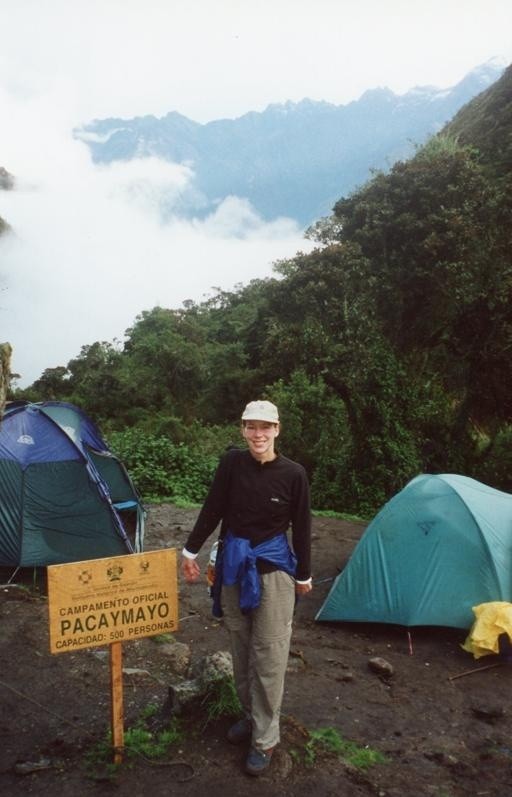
[241,400,280,425]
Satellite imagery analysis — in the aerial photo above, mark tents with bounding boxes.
[0,400,146,568]
[314,473,512,630]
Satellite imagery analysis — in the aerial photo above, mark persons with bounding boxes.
[182,400,312,776]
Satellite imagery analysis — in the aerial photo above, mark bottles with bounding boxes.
[206,540,219,586]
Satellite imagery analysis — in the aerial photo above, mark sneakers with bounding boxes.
[244,747,273,775]
[226,718,252,743]
